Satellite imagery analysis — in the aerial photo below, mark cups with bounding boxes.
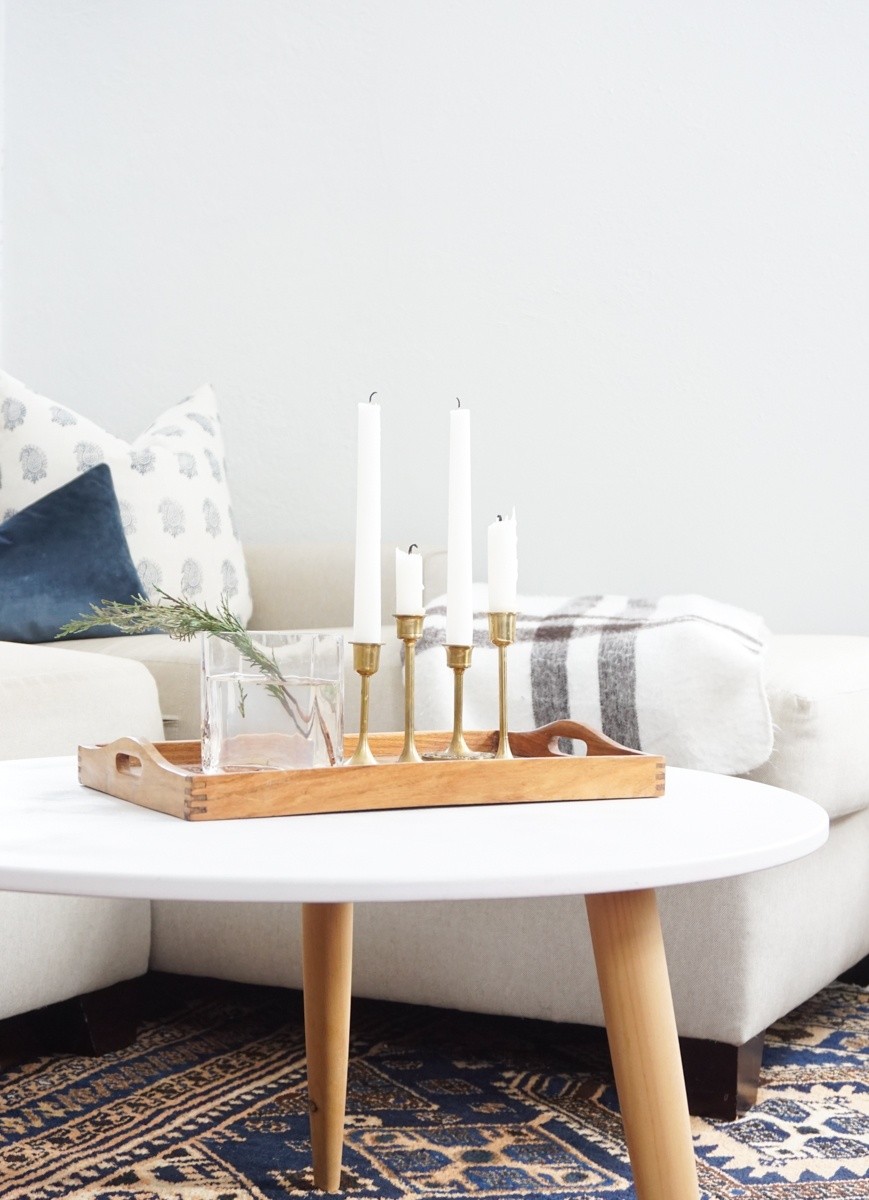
[200,627,346,775]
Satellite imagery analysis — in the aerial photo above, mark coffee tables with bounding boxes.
[0,754,829,1200]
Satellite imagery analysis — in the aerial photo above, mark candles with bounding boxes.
[447,397,473,644]
[354,392,381,641]
[396,544,423,615]
[487,505,519,613]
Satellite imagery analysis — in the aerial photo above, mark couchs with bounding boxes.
[0,552,869,1120]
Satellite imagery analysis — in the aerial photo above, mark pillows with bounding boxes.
[398,584,774,775]
[0,372,252,629]
[0,463,151,644]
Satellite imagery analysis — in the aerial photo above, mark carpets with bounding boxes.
[0,954,869,1200]
